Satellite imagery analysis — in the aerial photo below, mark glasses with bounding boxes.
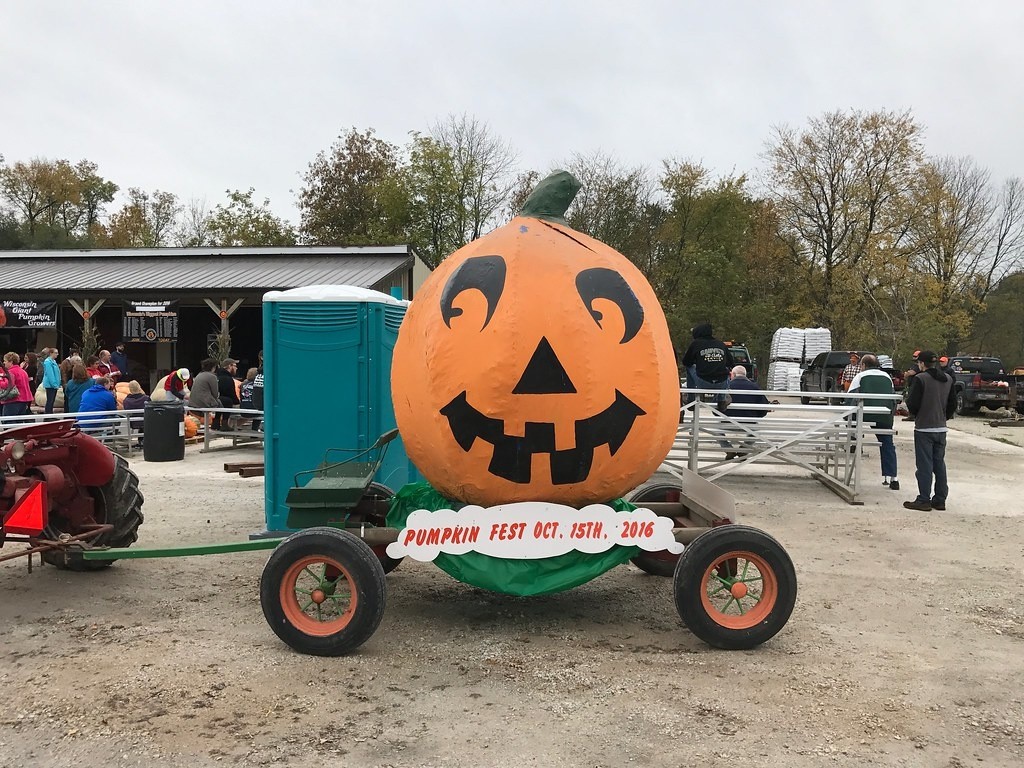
[232,364,238,368]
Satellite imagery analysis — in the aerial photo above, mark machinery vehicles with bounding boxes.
[0,286,799,658]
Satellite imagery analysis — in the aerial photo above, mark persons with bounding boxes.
[901,351,956,422]
[712,365,768,461]
[841,354,863,420]
[1,339,265,432]
[843,355,900,490]
[903,351,958,511]
[682,324,732,415]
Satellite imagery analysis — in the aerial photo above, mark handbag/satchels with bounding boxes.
[0,385,21,401]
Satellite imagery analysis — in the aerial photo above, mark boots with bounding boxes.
[220,419,233,432]
[211,418,221,431]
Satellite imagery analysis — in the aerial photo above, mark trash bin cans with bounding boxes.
[144,401,185,462]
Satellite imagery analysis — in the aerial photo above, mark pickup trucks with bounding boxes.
[799,351,906,405]
[723,341,759,384]
[947,357,1024,416]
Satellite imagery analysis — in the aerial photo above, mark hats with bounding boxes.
[912,350,921,357]
[221,358,239,367]
[115,342,126,347]
[849,352,861,360]
[911,350,937,363]
[938,357,948,362]
[177,368,190,381]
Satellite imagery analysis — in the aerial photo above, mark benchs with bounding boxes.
[653,389,903,505]
[0,406,265,459]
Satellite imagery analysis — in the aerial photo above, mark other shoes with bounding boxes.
[902,416,916,421]
[737,444,748,461]
[682,393,695,411]
[713,394,732,417]
[725,452,737,460]
[889,481,900,490]
[903,496,932,511]
[844,444,863,454]
[930,499,946,510]
